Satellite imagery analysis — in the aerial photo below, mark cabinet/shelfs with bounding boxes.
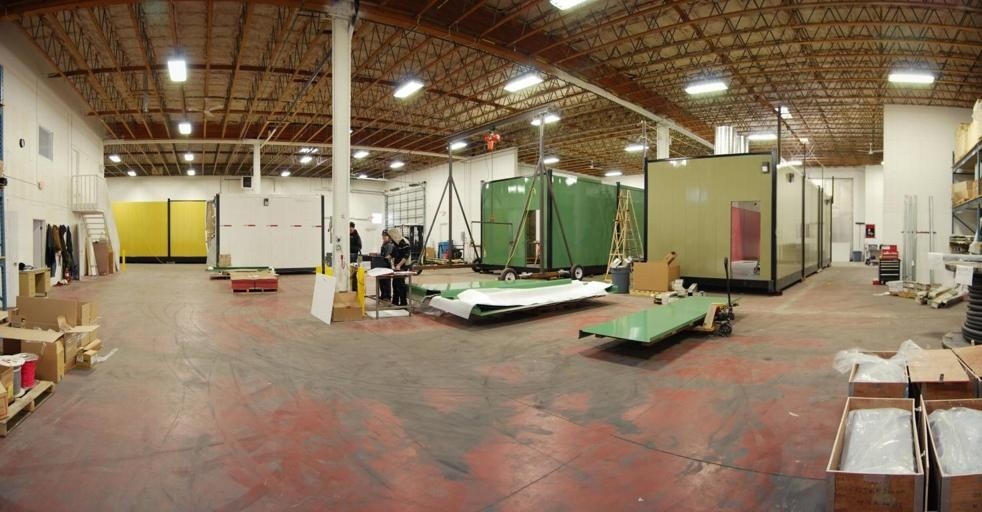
[951,138,982,254]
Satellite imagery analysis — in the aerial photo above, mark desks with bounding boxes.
[350,264,417,320]
[19,268,51,297]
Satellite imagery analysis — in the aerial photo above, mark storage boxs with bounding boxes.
[0,291,103,383]
[952,180,974,207]
[823,344,982,512]
[634,262,680,291]
[332,292,363,321]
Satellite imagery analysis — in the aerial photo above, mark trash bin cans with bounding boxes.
[851,251,862,262]
[610,267,630,293]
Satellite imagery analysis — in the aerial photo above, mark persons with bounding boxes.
[384,228,413,311]
[349,222,363,264]
[378,229,395,302]
